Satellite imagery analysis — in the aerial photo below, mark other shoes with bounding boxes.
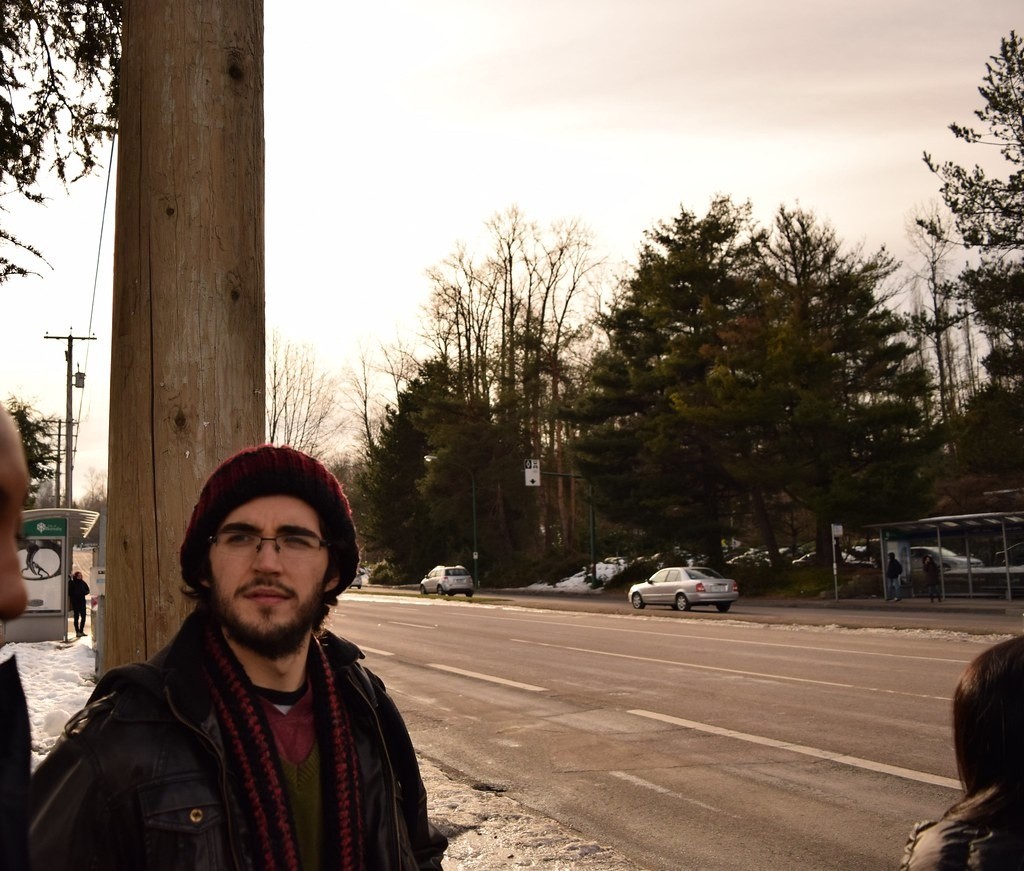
[77,631,87,637]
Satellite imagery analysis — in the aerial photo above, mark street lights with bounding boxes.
[424,455,479,590]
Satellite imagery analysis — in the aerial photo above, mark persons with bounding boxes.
[887,552,901,601]
[0,404,32,871]
[69,572,90,637]
[924,555,941,602]
[30,446,447,871]
[899,635,1024,871]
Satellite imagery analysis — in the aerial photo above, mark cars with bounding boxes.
[628,567,739,611]
[419,565,474,596]
[910,547,984,570]
[348,574,362,589]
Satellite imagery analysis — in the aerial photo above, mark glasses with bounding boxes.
[209,531,329,557]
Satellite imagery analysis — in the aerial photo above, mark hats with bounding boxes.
[180,445,359,604]
